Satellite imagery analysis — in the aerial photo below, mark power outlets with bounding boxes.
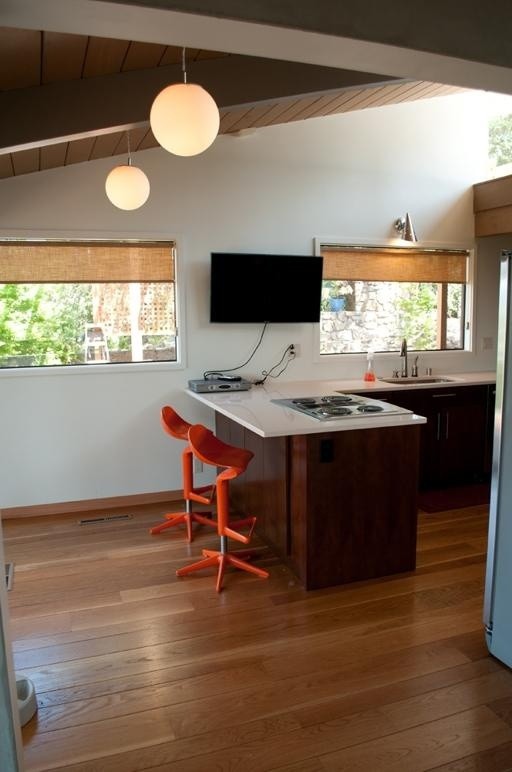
[284,342,297,358]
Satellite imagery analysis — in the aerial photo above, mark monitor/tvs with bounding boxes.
[210,252,323,323]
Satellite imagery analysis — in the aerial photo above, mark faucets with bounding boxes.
[400,338,408,377]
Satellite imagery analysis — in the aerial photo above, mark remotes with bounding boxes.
[218,375,241,381]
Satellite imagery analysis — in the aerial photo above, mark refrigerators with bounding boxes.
[482,249,511,671]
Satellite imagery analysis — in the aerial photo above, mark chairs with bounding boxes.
[150,402,224,544]
[175,423,271,599]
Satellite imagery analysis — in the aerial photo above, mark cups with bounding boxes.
[425,368,431,375]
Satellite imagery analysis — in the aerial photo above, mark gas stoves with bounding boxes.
[270,394,413,421]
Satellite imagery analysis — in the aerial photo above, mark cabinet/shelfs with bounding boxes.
[485,385,496,505]
[410,385,490,514]
[344,391,410,413]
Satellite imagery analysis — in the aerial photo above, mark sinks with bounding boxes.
[379,377,455,384]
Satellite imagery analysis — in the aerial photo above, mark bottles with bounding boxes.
[363,348,375,381]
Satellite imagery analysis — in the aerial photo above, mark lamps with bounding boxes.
[143,44,225,158]
[96,113,150,212]
[394,211,418,243]
